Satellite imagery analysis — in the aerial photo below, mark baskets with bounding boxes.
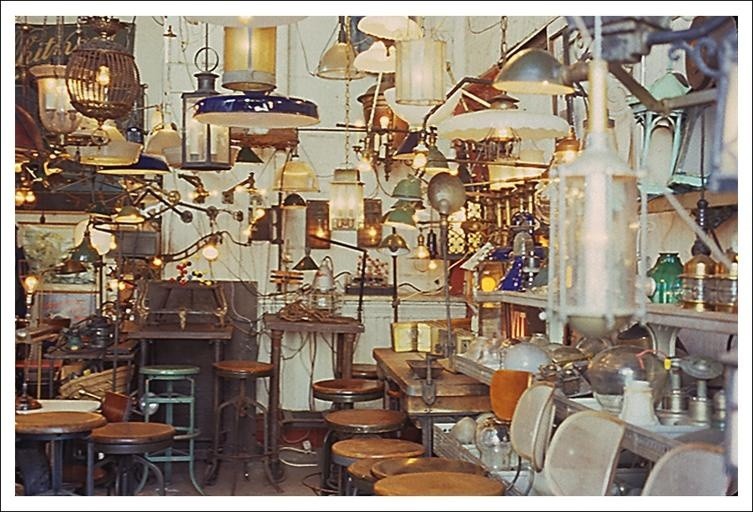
[58,362,131,402]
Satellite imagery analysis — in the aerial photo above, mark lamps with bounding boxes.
[14,13,737,334]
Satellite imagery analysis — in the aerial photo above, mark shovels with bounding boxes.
[421,355,435,406]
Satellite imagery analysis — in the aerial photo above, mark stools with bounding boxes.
[15,334,739,496]
